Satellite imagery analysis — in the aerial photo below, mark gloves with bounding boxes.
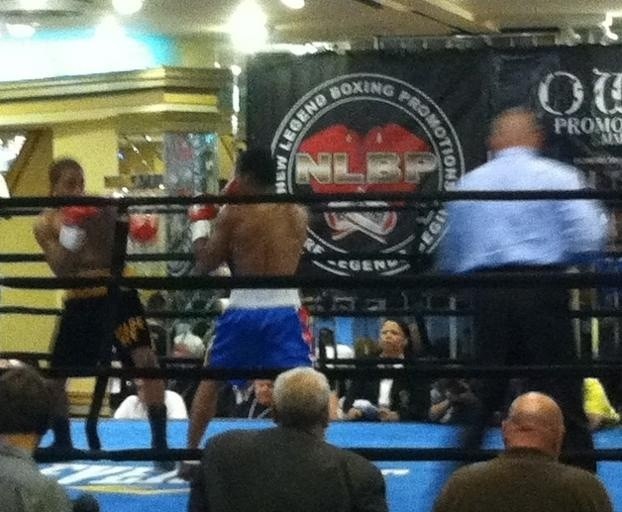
[130,215,159,243]
[218,177,241,196]
[62,204,100,224]
[187,200,217,220]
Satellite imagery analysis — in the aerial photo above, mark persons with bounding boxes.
[185,365,390,512]
[426,104,610,484]
[2,358,99,512]
[92,318,620,432]
[181,144,319,479]
[431,392,614,511]
[27,156,177,474]
